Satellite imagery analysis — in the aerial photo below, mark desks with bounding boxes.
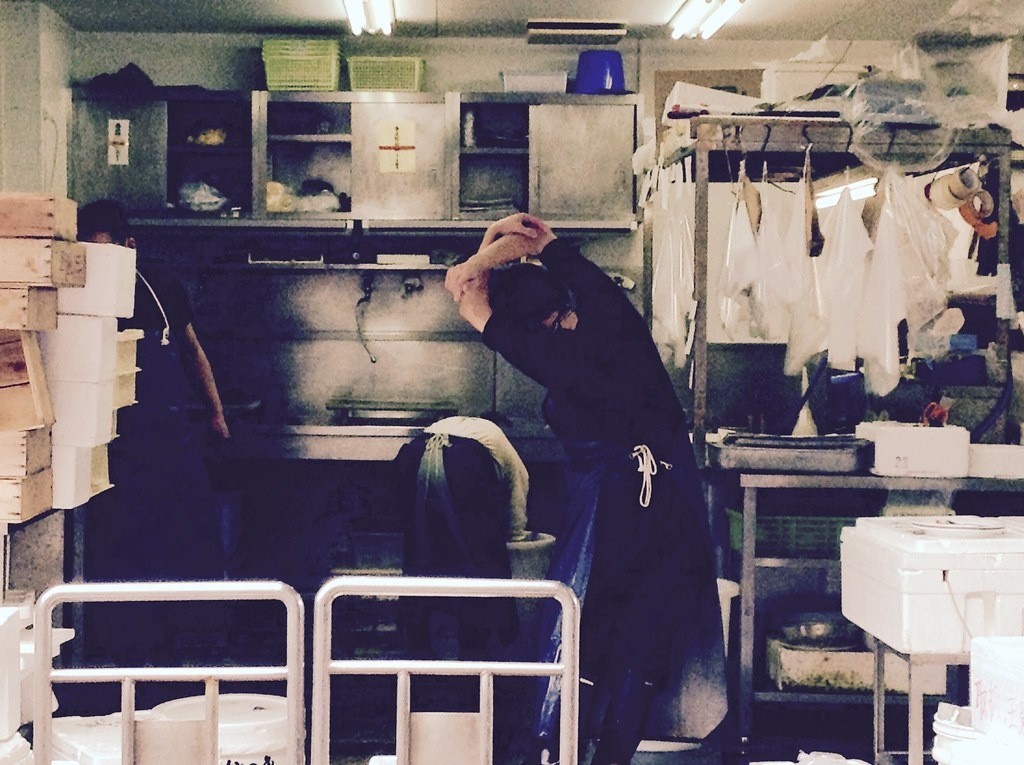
[735,474,1024,764]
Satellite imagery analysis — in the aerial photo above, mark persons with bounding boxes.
[390,416,529,705]
[76,199,230,581]
[446,213,727,765]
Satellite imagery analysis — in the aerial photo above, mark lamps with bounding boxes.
[666,0,745,41]
[341,0,395,38]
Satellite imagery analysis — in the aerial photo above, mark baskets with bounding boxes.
[346,55,427,90]
[726,507,858,553]
[262,37,344,92]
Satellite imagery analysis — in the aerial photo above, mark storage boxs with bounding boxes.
[259,40,425,91]
[0,196,152,764]
[661,81,766,131]
[725,506,877,558]
[839,516,1024,654]
[855,420,974,478]
[500,66,574,92]
[969,638,1023,742]
[969,442,1022,479]
[766,638,949,695]
[758,65,871,119]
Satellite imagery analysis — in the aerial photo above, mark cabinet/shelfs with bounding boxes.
[254,85,444,229]
[69,93,253,220]
[447,87,630,224]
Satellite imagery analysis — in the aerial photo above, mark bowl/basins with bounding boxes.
[779,612,858,647]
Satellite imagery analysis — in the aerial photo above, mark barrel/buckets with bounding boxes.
[932,705,1023,765]
[153,693,290,765]
[574,51,624,94]
